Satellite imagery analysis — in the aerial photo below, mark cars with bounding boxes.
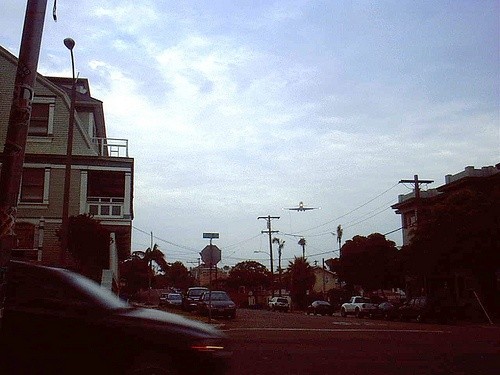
[159,285,236,319]
[0,255,234,375]
[306,300,334,316]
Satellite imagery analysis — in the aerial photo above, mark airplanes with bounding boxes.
[281,201,320,212]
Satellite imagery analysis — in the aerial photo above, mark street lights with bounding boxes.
[59,37,77,269]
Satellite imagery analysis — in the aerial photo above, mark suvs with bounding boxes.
[340,288,435,324]
[268,296,290,314]
